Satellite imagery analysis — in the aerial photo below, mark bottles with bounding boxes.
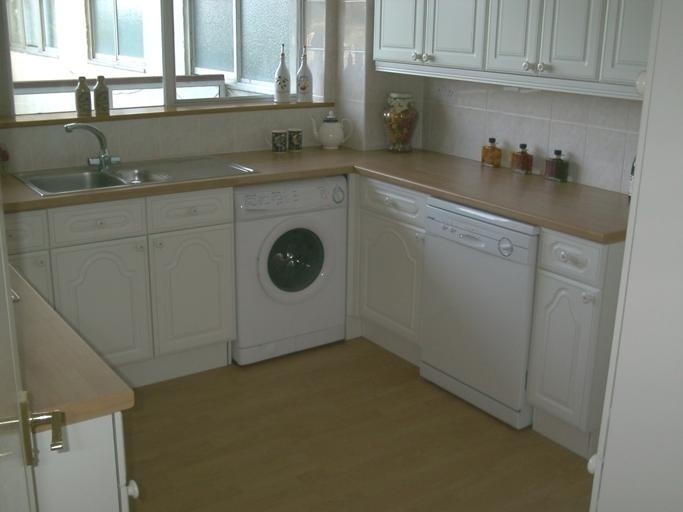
[75,76,91,117]
[479,138,502,168]
[544,149,569,183]
[294,46,312,103]
[273,45,290,104]
[511,144,532,175]
[92,76,109,118]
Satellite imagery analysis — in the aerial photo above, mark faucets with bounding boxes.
[64,123,115,169]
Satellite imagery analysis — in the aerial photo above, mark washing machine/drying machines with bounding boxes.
[230,175,347,367]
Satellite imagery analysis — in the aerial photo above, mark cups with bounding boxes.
[270,129,303,153]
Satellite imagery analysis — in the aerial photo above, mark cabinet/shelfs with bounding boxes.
[9,263,139,512]
[358,175,430,349]
[589,0,683,512]
[373,0,489,71]
[526,227,609,434]
[485,0,606,82]
[5,209,54,307]
[598,0,664,87]
[48,186,238,368]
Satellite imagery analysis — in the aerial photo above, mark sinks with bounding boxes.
[28,174,125,194]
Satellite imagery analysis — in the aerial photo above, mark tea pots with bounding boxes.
[308,110,354,151]
